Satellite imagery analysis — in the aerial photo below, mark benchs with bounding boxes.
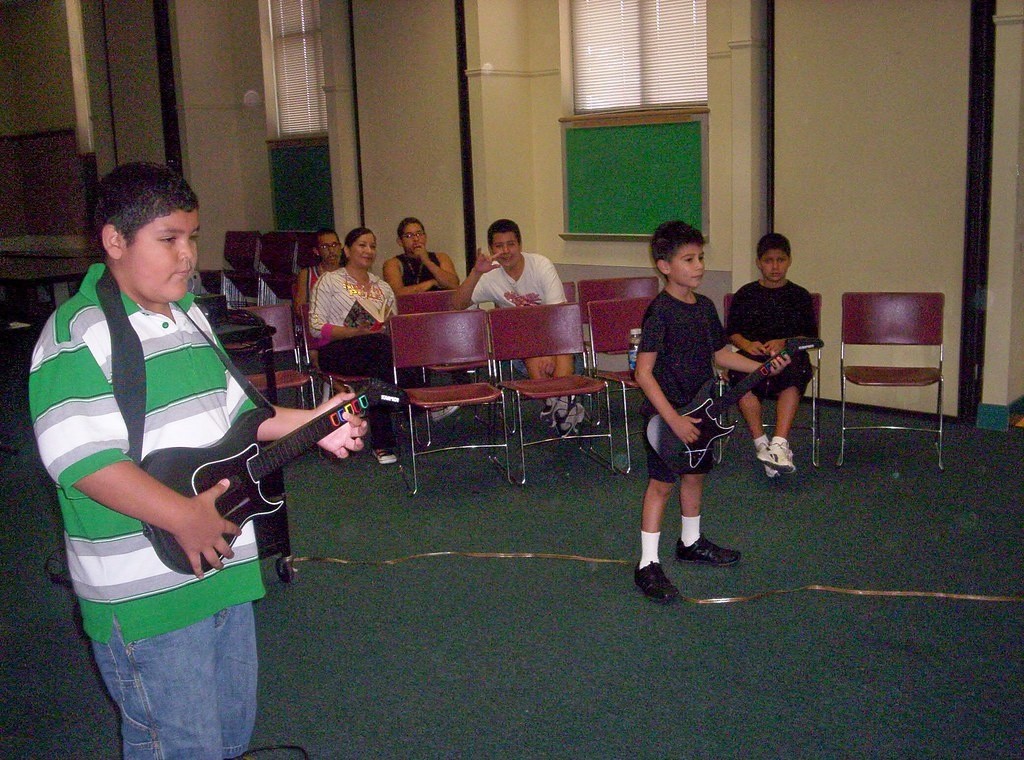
[300,302,376,406]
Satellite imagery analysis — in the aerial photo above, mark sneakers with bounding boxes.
[634,560,679,604]
[757,441,795,478]
[539,392,584,438]
[677,533,742,567]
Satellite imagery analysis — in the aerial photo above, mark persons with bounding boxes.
[380,216,459,390]
[450,219,584,437]
[28,159,368,760]
[290,229,345,394]
[633,220,791,602]
[309,227,461,464]
[726,233,817,480]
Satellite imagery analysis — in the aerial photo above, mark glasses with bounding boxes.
[401,231,425,237]
[317,242,341,251]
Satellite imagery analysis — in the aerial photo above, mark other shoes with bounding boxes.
[430,406,459,422]
[373,447,396,464]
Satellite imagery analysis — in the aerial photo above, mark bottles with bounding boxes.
[628,328,642,380]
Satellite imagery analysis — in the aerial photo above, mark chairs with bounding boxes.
[191,230,320,306]
[387,310,515,495]
[488,305,613,483]
[838,291,947,471]
[393,288,488,444]
[721,293,825,468]
[578,276,665,322]
[587,295,661,476]
[235,307,313,421]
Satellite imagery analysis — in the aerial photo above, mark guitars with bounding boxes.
[139,379,409,576]
[646,336,825,474]
